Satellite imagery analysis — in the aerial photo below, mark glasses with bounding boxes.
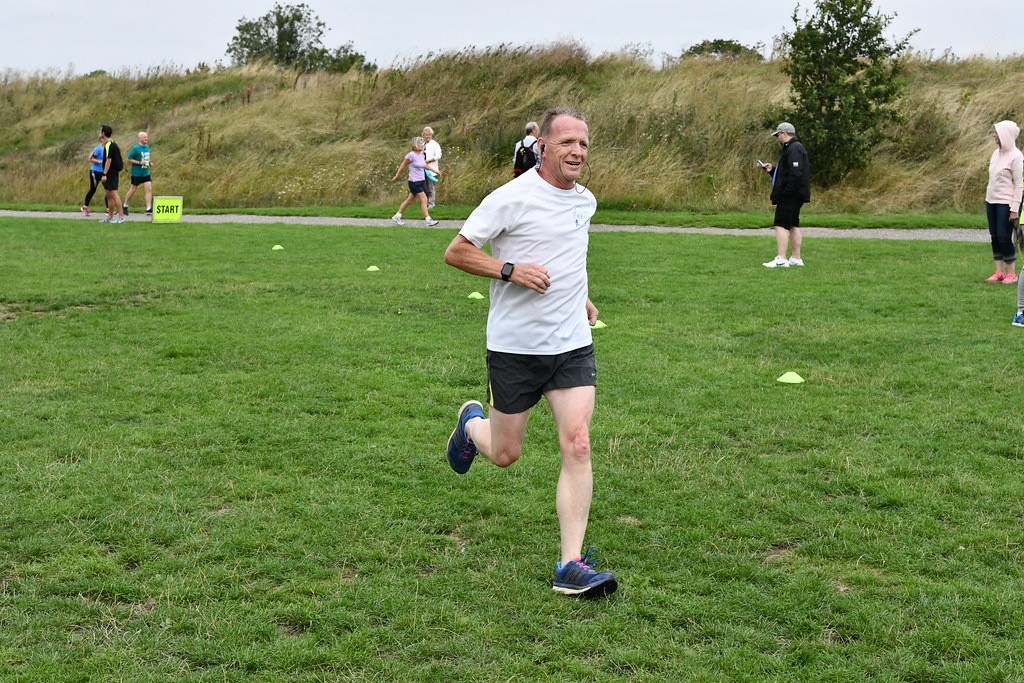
[776,132,783,137]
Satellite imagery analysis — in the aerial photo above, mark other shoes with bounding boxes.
[122,203,128,216]
[146,208,153,215]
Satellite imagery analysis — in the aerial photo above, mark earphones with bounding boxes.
[541,144,544,155]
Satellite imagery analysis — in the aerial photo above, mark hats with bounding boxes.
[772,122,795,137]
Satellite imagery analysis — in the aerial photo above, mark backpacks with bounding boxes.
[514,140,537,177]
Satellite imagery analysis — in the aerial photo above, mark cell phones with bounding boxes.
[757,160,765,168]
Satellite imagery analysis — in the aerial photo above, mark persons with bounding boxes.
[81,125,125,223]
[762,123,810,267]
[391,126,442,225]
[444,106,619,600]
[984,120,1024,327]
[513,122,540,164]
[122,131,154,216]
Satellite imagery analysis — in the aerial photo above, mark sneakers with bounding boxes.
[789,255,805,266]
[427,219,439,226]
[80,206,91,216]
[1001,274,1019,284]
[1011,309,1024,327]
[392,214,405,225]
[106,211,118,216]
[988,271,1007,282]
[110,216,126,224]
[99,216,114,223]
[553,543,618,599]
[447,400,486,474]
[762,255,789,268]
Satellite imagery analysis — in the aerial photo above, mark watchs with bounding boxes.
[501,262,514,282]
